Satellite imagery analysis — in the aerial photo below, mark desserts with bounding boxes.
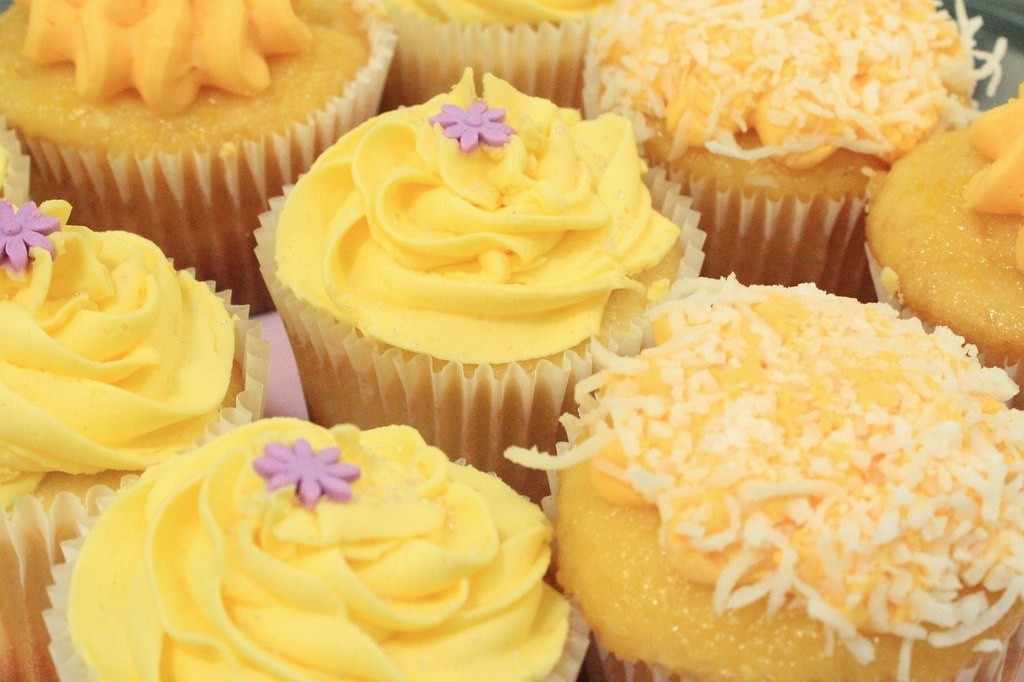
[2,0,1024,682]
